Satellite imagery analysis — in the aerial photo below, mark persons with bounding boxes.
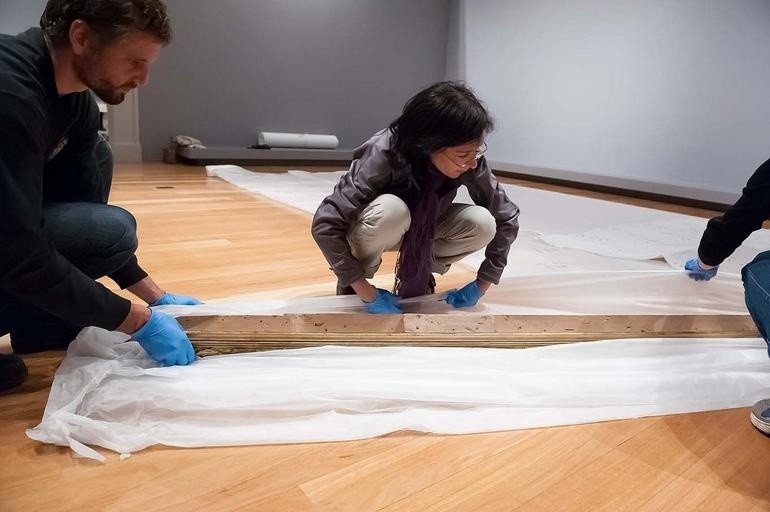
[310,79,520,311]
[684,157,770,435]
[0,1,206,392]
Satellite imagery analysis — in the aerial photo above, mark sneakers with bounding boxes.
[337,281,356,295]
[750,398,770,434]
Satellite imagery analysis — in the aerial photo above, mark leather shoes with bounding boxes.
[0,354,28,390]
[11,332,78,354]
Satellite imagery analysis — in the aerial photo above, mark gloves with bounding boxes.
[131,307,195,366]
[447,281,483,308]
[685,257,719,281]
[361,285,404,314]
[149,291,205,306]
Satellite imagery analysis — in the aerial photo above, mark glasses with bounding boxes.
[441,140,487,169]
